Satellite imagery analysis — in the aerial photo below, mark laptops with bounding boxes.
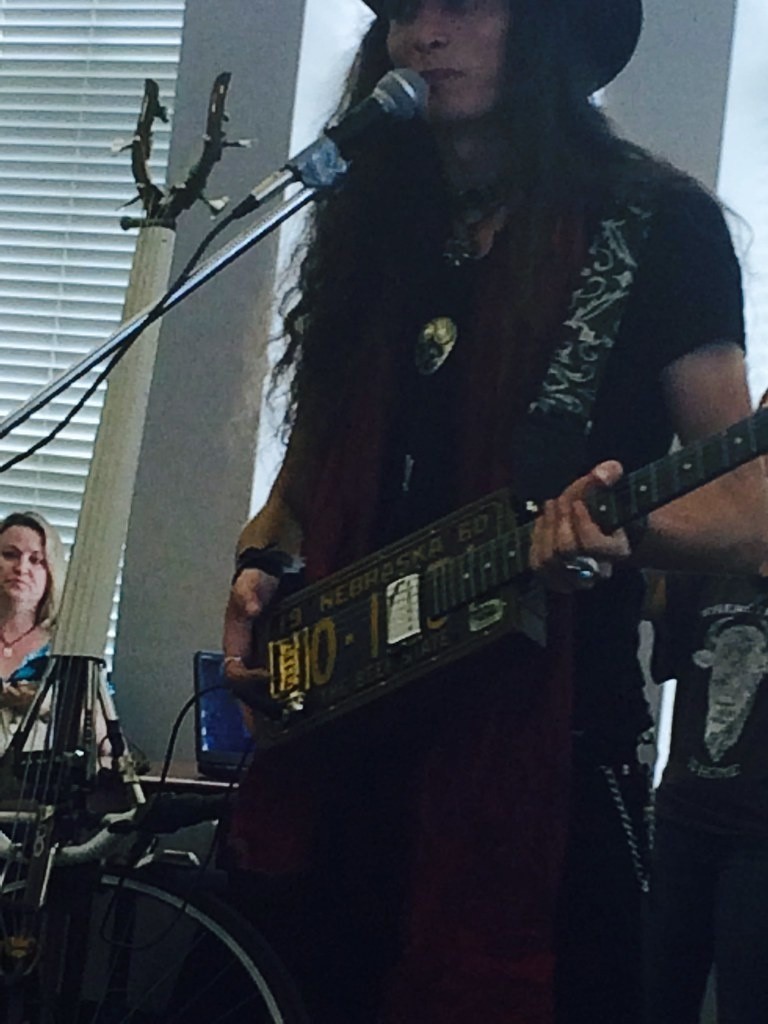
[195,651,254,782]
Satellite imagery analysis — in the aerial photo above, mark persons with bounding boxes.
[219,0,768,1024]
[0,512,129,772]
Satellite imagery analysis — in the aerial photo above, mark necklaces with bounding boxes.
[415,168,517,375]
[0,621,38,657]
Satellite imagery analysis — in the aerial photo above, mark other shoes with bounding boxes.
[1,907,48,979]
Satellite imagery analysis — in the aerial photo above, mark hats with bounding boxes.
[363,0,643,97]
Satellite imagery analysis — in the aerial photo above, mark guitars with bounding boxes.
[1,63,290,1024]
[252,400,768,757]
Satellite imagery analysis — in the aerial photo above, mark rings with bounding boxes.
[223,656,241,668]
[566,556,599,579]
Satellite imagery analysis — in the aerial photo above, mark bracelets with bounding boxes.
[231,544,284,585]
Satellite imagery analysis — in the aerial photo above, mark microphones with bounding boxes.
[235,67,431,219]
